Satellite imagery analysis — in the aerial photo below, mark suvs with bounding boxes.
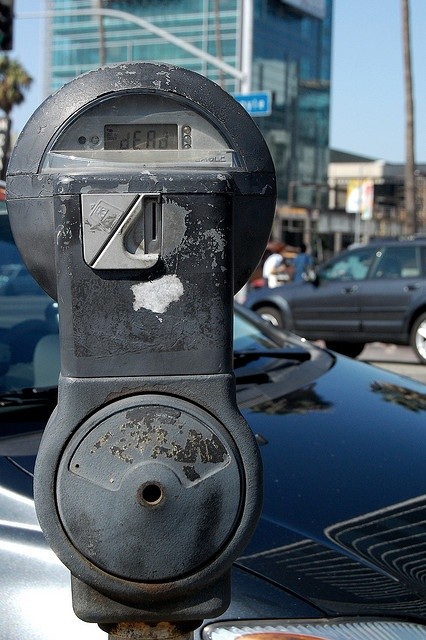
[244,240,425,363]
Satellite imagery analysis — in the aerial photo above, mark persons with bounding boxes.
[288,241,315,284]
[261,237,289,287]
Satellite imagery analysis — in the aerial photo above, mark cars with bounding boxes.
[4,243,425,640]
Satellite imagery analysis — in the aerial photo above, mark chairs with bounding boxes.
[33,334,59,387]
[401,267,418,276]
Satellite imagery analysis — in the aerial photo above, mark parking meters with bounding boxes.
[4,60,282,632]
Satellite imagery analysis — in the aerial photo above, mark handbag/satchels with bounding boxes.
[277,273,289,281]
